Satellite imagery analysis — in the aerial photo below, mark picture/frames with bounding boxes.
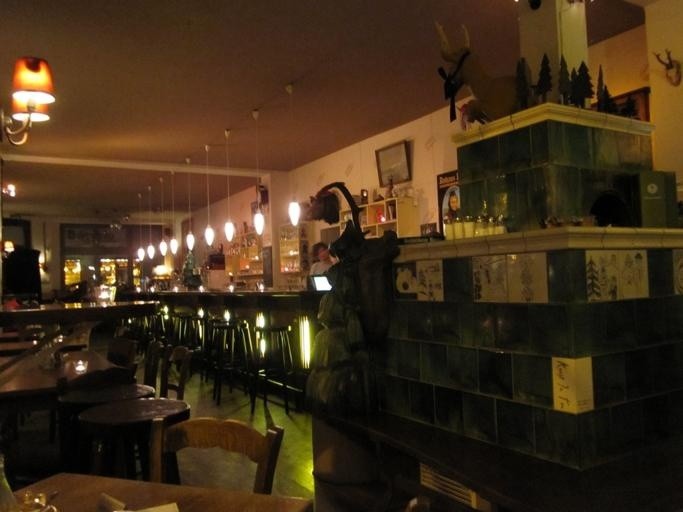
[374,139,412,188]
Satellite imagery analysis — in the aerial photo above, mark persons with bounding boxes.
[308,242,333,275]
[445,192,459,219]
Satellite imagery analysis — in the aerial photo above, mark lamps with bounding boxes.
[1,183,16,201]
[136,76,301,263]
[0,57,58,148]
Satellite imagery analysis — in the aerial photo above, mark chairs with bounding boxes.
[159,410,284,494]
[0,318,191,484]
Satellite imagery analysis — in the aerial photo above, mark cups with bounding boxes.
[387,203,394,220]
[73,360,88,375]
[420,222,437,241]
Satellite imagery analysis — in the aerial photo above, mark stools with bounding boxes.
[131,308,294,416]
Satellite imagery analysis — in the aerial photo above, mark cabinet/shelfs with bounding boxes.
[340,197,421,243]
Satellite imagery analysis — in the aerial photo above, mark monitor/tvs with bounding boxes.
[312,274,332,293]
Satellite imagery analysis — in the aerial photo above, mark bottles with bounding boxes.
[384,173,393,200]
[225,220,295,260]
[0,454,22,512]
[443,215,507,242]
